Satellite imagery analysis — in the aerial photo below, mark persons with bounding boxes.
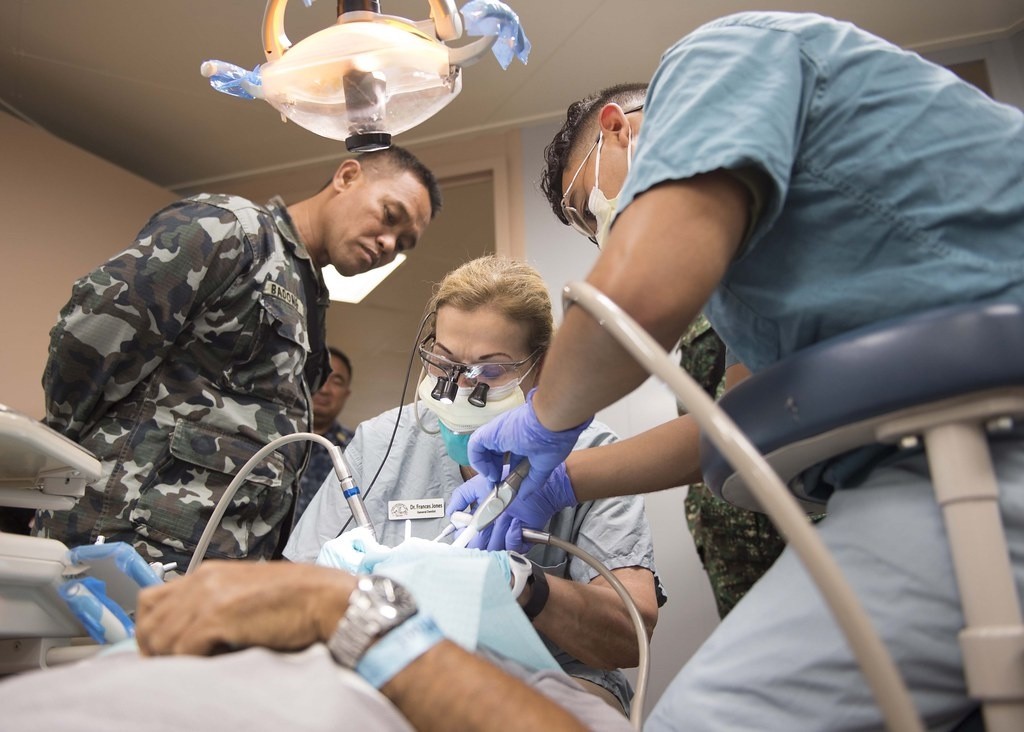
[138,558,632,732]
[30,145,442,580]
[283,253,668,720]
[291,347,356,534]
[446,10,1024,732]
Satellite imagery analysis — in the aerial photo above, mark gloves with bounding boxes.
[467,387,595,502]
[445,460,579,554]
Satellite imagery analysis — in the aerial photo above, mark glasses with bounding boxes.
[560,103,644,244]
[419,333,541,387]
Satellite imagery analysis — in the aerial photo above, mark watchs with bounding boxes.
[328,573,419,671]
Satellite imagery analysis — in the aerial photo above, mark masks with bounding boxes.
[417,358,541,432]
[589,123,631,249]
